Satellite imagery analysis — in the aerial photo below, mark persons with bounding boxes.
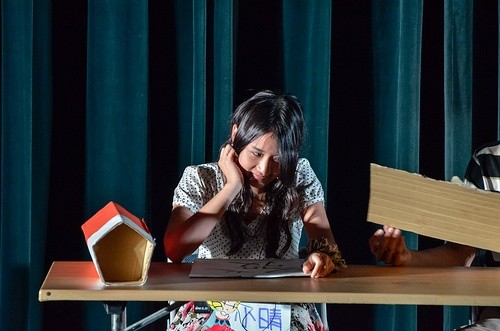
[368,141,500,331]
[164,90,340,331]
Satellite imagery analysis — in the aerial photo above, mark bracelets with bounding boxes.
[306,236,348,271]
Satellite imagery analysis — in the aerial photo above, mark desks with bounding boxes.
[38,260,500,331]
